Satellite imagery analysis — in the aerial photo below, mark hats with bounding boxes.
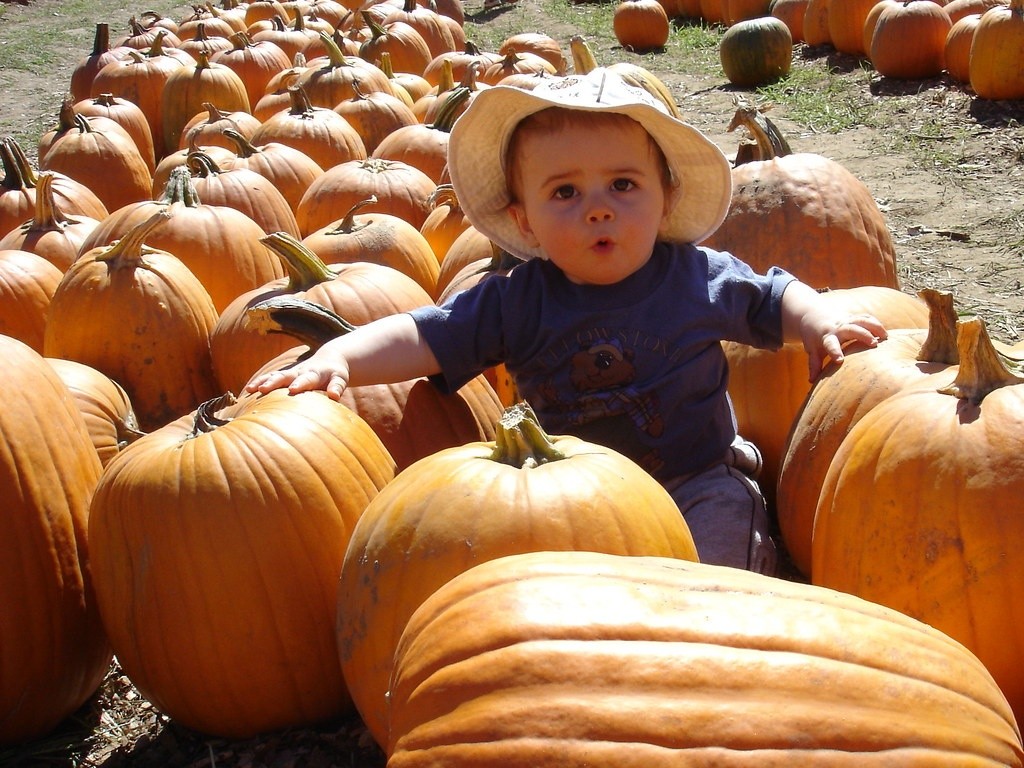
[447,67,732,263]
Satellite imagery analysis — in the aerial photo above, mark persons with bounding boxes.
[247,66,889,583]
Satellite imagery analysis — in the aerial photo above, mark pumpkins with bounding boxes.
[0,1,1024,768]
